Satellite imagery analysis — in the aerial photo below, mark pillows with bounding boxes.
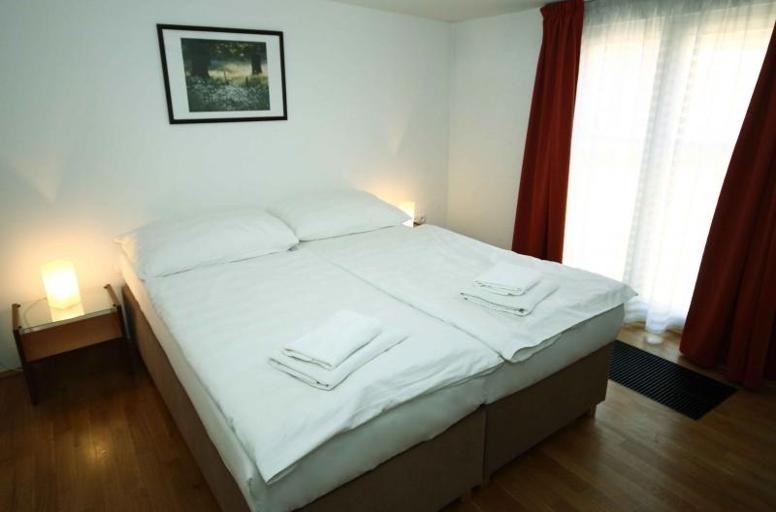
[267,190,412,241]
[111,205,300,278]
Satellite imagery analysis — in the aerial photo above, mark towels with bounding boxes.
[285,311,376,368]
[473,261,542,296]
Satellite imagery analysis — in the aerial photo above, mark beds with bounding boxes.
[121,222,638,512]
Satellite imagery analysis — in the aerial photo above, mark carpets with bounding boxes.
[608,337,738,421]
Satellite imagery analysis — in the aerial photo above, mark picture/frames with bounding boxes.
[154,22,287,125]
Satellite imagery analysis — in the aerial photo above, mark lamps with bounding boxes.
[40,257,82,310]
[398,200,415,228]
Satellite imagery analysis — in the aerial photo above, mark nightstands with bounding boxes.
[413,222,420,228]
[12,283,134,405]
